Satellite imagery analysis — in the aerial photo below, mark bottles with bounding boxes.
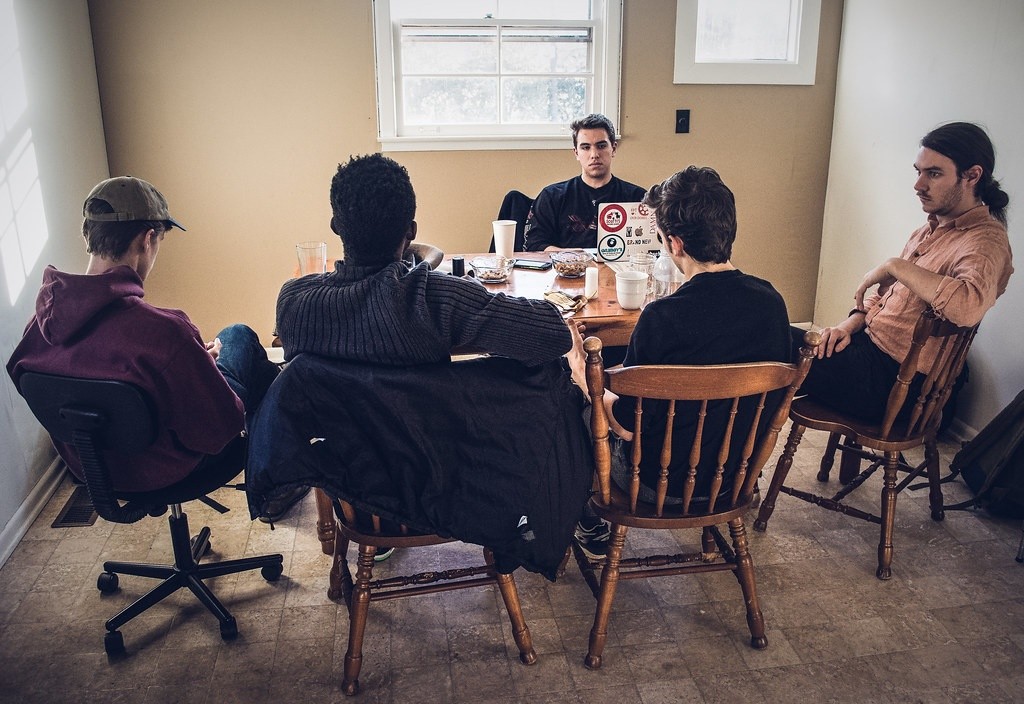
[630,254,658,296]
[652,250,685,300]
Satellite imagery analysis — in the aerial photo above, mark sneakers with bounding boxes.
[574,521,610,559]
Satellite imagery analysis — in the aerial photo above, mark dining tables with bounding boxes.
[295,251,681,555]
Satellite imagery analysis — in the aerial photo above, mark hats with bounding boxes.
[82,176,187,231]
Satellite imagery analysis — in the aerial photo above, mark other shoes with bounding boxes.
[372,544,395,562]
[260,483,312,522]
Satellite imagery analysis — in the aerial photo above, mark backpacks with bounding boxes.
[949,388,1024,520]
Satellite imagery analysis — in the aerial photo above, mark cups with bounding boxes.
[615,272,649,310]
[492,220,517,258]
[296,242,328,276]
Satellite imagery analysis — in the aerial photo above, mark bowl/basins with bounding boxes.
[469,254,516,284]
[549,251,594,279]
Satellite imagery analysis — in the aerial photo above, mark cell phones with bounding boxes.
[514,259,552,271]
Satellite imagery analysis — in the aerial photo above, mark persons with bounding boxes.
[5,175,312,524]
[750,122,1014,510]
[521,115,647,251]
[276,153,572,562]
[574,166,794,561]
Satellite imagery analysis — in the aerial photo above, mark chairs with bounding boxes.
[753,306,981,580]
[19,372,283,656]
[325,496,536,696]
[555,331,822,671]
